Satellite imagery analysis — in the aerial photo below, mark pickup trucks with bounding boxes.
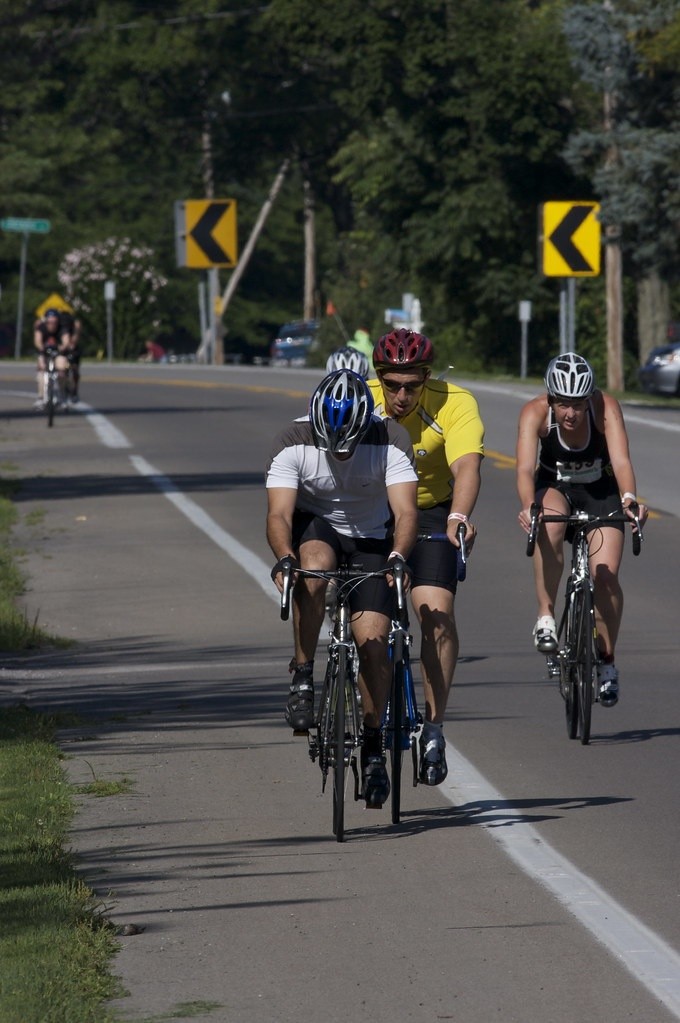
[270,321,322,368]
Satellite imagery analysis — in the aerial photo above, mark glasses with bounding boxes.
[382,375,426,391]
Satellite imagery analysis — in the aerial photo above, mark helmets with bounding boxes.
[545,351,594,402]
[309,369,375,455]
[373,328,433,375]
[326,346,370,379]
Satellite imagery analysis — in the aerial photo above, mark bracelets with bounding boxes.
[621,492,635,504]
[388,551,405,563]
[447,512,468,523]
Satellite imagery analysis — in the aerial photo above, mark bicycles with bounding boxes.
[382,526,467,827]
[526,499,643,745]
[40,349,72,428]
[275,558,406,845]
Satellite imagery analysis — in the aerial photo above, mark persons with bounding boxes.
[516,353,649,707]
[33,309,80,412]
[266,370,420,810]
[138,340,170,366]
[358,329,484,788]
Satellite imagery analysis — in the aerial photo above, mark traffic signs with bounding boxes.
[1,219,51,232]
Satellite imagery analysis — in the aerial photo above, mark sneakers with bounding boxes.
[598,665,618,707]
[418,731,447,786]
[285,661,315,730]
[532,615,558,652]
[362,754,390,806]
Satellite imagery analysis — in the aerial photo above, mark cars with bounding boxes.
[639,345,679,398]
